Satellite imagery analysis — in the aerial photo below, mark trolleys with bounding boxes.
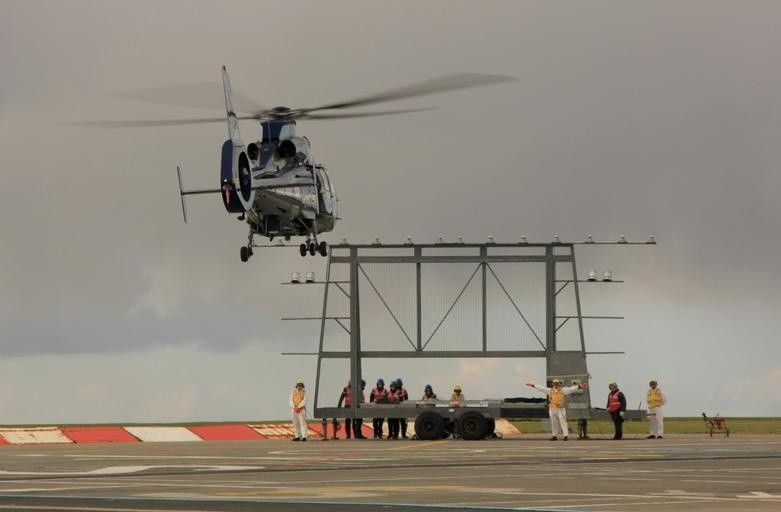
[705,414,730,439]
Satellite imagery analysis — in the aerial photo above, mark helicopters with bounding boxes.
[51,64,518,261]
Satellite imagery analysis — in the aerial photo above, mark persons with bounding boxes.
[337,379,367,439]
[606,381,626,440]
[421,384,437,400]
[526,378,587,441]
[289,379,308,441]
[385,381,402,440]
[449,383,465,400]
[646,379,667,439]
[370,378,390,438]
[395,378,408,439]
